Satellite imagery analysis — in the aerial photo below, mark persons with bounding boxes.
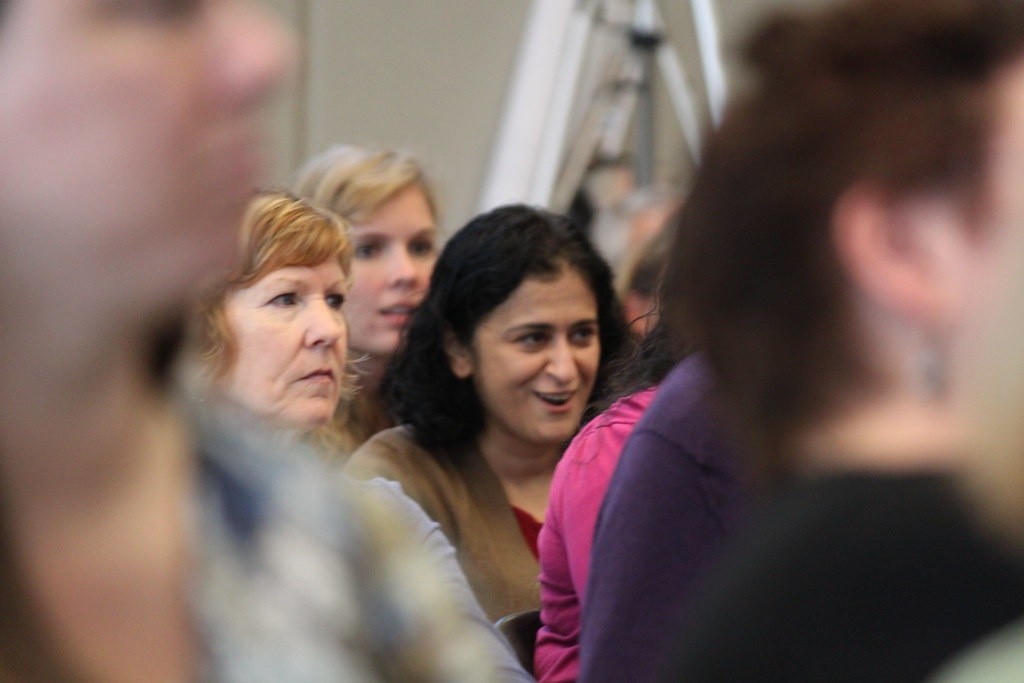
[0,0,1024,683]
